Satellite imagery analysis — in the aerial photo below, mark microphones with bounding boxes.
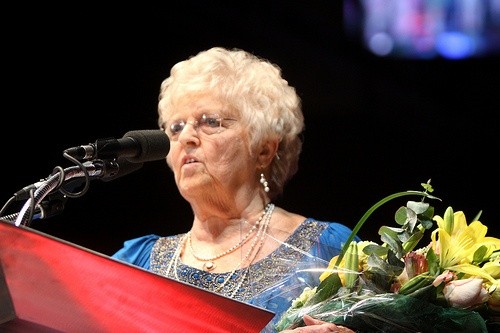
[14,175,97,201]
[65,130,169,163]
[19,199,64,221]
[85,160,144,182]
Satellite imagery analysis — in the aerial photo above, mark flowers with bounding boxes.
[261,180,498,332]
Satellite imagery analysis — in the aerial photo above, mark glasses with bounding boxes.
[165,113,281,161]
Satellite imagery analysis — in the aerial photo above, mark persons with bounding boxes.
[109,47,363,333]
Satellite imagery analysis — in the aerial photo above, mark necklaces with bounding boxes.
[164,203,274,298]
